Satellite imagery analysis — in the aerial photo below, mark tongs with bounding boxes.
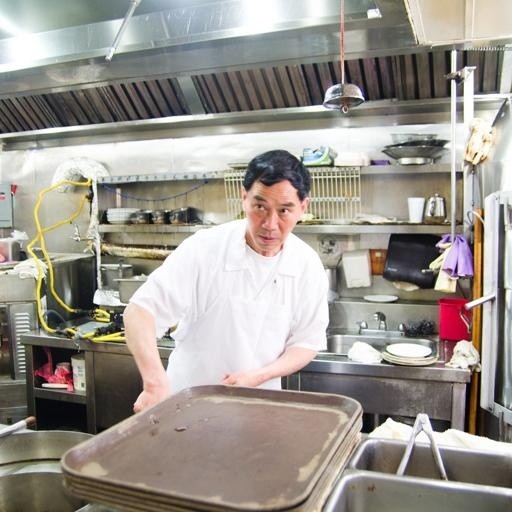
[396,412,447,481]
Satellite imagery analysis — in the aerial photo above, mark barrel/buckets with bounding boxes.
[71,354,86,391]
[437,299,471,341]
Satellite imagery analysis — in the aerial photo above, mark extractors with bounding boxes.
[0,1,512,145]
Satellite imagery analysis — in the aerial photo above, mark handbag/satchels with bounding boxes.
[382,234,442,289]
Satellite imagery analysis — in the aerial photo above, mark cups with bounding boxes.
[407,197,425,223]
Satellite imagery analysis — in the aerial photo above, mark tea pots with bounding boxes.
[425,193,446,224]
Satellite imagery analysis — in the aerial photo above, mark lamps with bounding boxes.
[318,0,368,113]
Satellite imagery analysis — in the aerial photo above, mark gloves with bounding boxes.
[431,234,475,294]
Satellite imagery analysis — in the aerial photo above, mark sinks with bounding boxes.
[316,335,440,356]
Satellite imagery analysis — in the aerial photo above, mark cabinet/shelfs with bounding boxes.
[90,158,475,235]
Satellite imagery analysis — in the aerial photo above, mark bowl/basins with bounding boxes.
[135,207,192,224]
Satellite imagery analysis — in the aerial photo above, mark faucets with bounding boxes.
[373,312,387,330]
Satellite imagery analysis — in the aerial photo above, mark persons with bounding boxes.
[123,149,329,413]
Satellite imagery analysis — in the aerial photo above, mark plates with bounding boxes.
[381,133,451,165]
[106,208,138,225]
[382,343,438,366]
[364,295,399,302]
